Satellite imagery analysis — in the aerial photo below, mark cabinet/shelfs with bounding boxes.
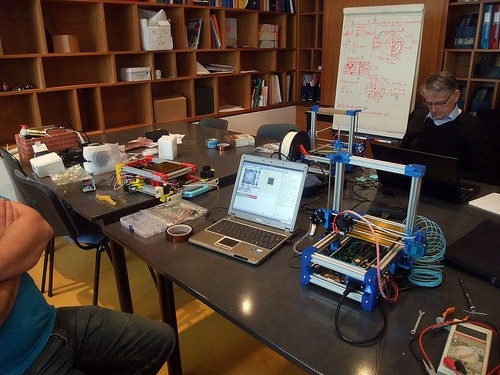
[0,0,325,131]
[439,0,500,111]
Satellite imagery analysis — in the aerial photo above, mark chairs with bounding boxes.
[0,143,112,306]
[255,123,298,140]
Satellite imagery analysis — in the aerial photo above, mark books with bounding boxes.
[218,105,245,112]
[210,14,222,49]
[186,18,203,49]
[158,0,294,14]
[203,63,236,74]
[251,74,290,108]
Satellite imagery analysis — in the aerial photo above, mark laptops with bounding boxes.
[188,154,309,266]
[369,141,480,205]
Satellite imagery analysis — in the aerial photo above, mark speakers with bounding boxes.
[84,143,117,175]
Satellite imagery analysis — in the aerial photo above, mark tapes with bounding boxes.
[166,223,193,243]
[216,143,230,151]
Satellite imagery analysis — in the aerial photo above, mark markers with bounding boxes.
[355,136,367,140]
[375,139,391,144]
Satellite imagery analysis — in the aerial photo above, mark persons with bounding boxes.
[0,195,176,375]
[399,70,493,182]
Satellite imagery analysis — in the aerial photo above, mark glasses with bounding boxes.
[423,88,458,106]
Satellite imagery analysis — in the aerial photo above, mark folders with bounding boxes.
[490,4,500,49]
[479,4,493,49]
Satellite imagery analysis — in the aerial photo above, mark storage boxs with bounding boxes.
[120,66,152,81]
[153,95,187,123]
[140,18,173,52]
[45,19,81,52]
[17,126,78,158]
[30,152,66,178]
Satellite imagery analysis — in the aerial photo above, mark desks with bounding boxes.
[20,118,500,375]
